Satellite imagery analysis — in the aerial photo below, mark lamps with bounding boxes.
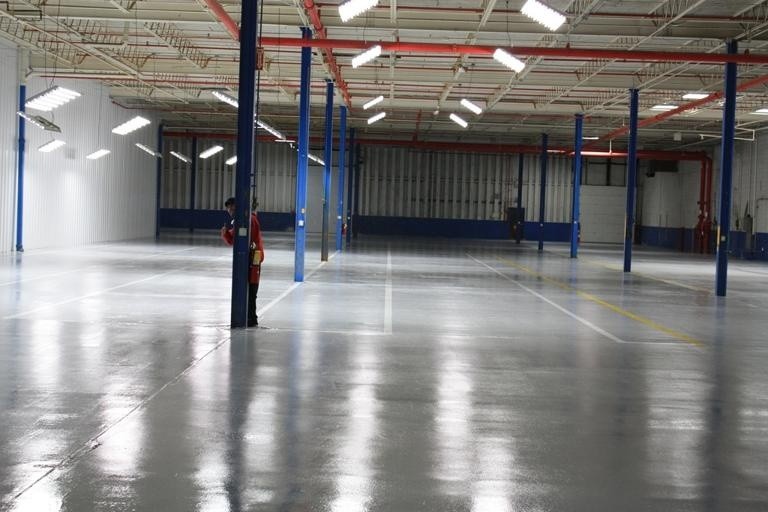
[169,151,192,163]
[112,116,151,136]
[210,91,238,109]
[276,141,295,142]
[307,153,324,165]
[86,148,111,159]
[339,0,567,129]
[19,111,62,134]
[38,140,65,152]
[136,144,162,158]
[226,156,237,164]
[24,87,83,111]
[200,145,223,159]
[257,121,286,140]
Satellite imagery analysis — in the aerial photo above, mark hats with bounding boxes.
[225,198,235,207]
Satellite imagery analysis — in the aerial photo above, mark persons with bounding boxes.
[222,197,264,328]
